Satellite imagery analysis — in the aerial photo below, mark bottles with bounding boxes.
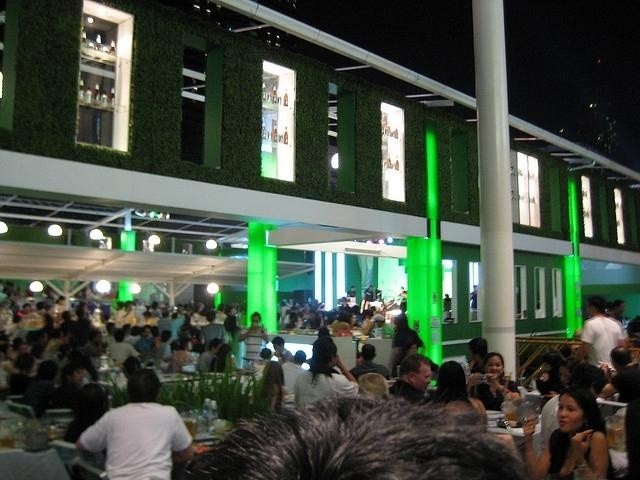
[382,123,400,171]
[80,30,117,107]
[201,397,218,432]
[262,82,291,146]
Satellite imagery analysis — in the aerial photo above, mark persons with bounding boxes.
[523,384,616,480]
[78,368,194,480]
[623,315,640,480]
[185,396,528,479]
[0,282,640,443]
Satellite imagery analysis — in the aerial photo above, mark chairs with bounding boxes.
[48,440,105,480]
[44,409,73,423]
[6,400,35,419]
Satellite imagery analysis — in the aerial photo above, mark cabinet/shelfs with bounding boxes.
[80,45,116,113]
[382,134,401,177]
[262,99,290,148]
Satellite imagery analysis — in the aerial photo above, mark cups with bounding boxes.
[184,418,197,436]
